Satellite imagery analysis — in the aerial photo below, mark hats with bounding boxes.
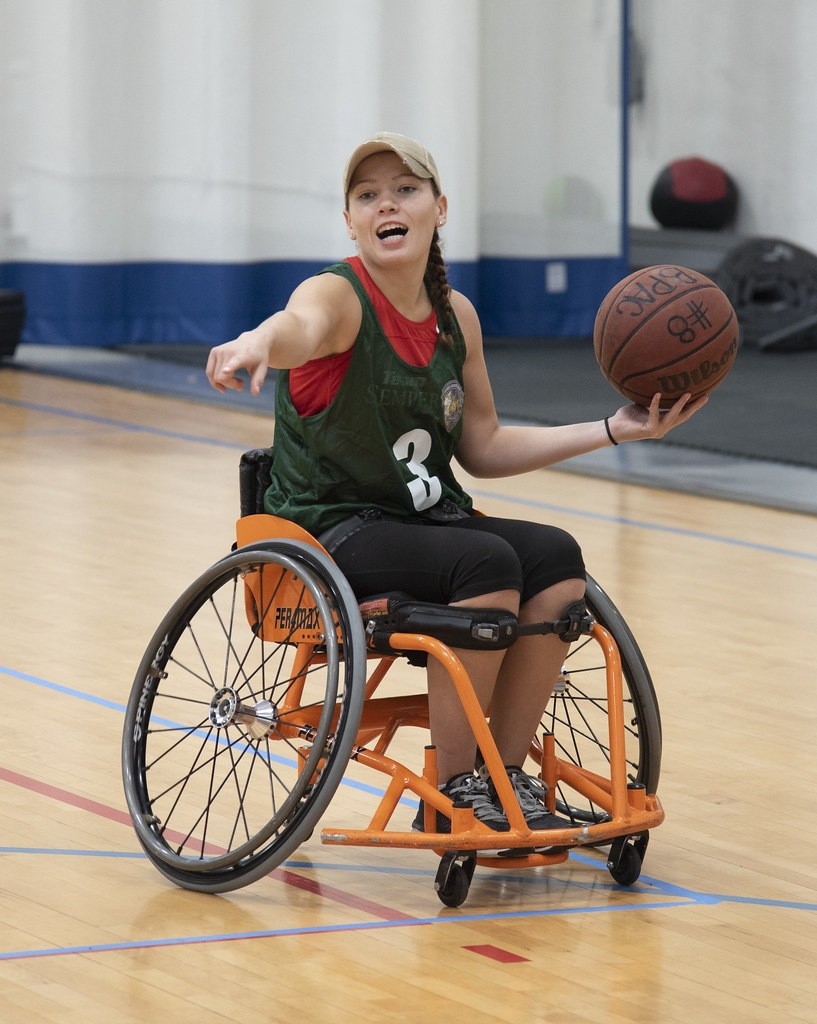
[342,130,441,197]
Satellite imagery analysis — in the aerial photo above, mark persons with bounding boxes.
[206,133,710,858]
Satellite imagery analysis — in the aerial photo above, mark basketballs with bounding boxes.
[593,265,740,406]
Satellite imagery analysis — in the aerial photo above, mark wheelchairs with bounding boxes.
[121,448,665,909]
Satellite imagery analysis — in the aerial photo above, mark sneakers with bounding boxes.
[476,764,584,852]
[410,772,535,857]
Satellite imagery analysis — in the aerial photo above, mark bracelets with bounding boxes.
[604,417,618,446]
